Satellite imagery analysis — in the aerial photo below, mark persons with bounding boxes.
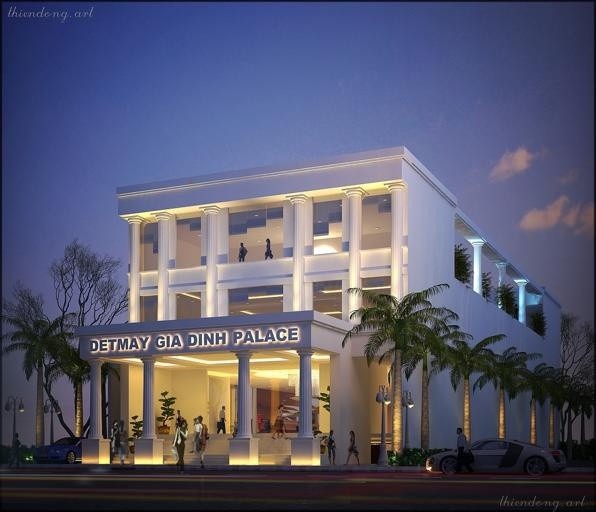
[192,416,209,469]
[264,237,273,260]
[109,420,126,465]
[272,405,287,440]
[216,406,227,434]
[8,432,22,469]
[343,430,362,466]
[173,419,189,474]
[176,410,185,426]
[238,242,245,262]
[325,429,337,465]
[454,426,469,474]
